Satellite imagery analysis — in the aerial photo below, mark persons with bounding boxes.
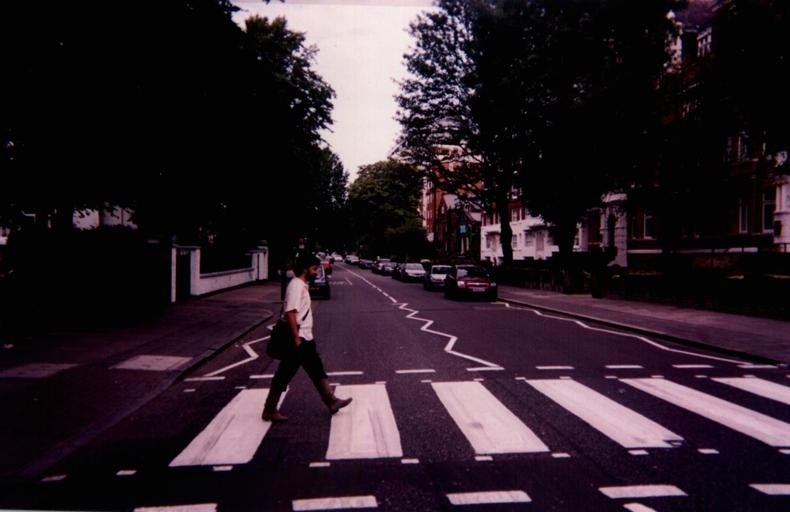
[261,254,352,422]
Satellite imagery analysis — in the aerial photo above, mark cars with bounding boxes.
[280,254,343,300]
[344,255,498,301]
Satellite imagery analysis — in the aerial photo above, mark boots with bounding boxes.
[262,389,288,423]
[314,378,353,414]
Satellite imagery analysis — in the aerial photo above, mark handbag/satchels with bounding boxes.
[266,319,300,360]
